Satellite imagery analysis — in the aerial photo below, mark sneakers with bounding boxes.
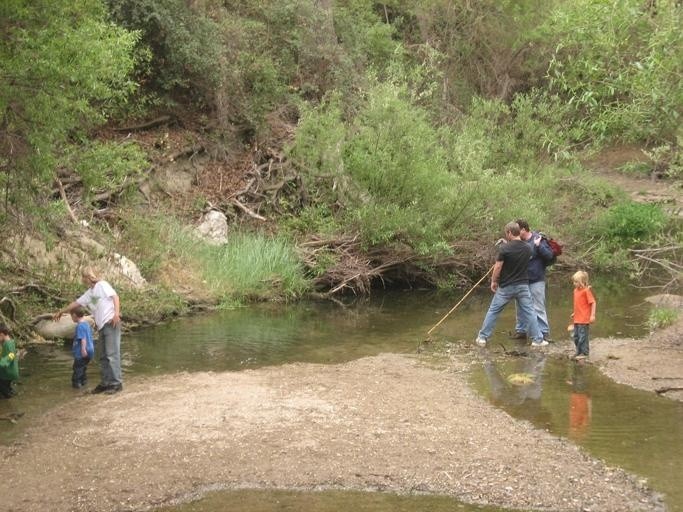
[531,332,552,347]
[92,384,122,395]
[513,333,526,339]
[476,337,487,344]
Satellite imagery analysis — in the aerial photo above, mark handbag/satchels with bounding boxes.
[540,234,562,256]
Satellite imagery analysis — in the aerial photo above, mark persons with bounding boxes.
[482,352,547,421]
[475,221,550,346]
[508,218,556,343]
[0,323,19,399]
[71,307,94,388]
[568,270,596,360]
[566,365,594,440]
[51,265,122,396]
